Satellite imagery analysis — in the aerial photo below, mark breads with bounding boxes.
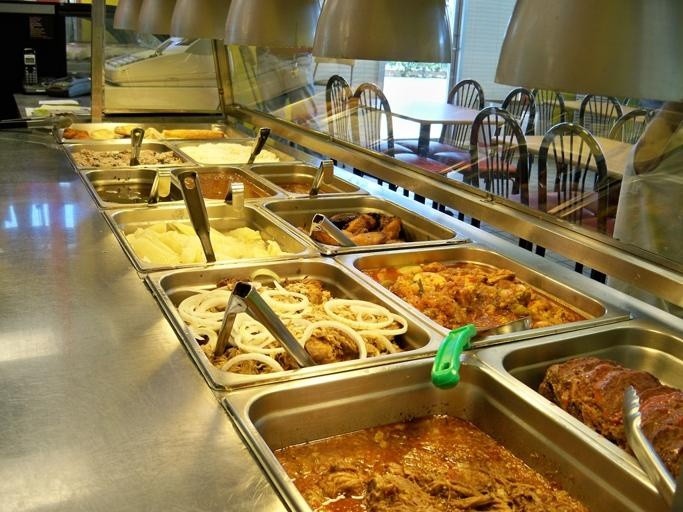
[61,125,162,141]
[159,128,227,139]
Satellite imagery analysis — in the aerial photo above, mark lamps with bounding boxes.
[137,0,176,36]
[494,0,683,106]
[171,0,231,41]
[313,0,452,65]
[223,1,321,48]
[113,1,139,33]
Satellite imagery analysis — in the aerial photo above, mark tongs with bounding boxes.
[214,278,320,368]
[302,213,357,248]
[616,379,683,512]
[145,165,173,206]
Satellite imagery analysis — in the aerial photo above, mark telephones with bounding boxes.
[22,48,48,95]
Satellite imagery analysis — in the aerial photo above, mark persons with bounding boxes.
[254,46,331,160]
[605,103,681,316]
